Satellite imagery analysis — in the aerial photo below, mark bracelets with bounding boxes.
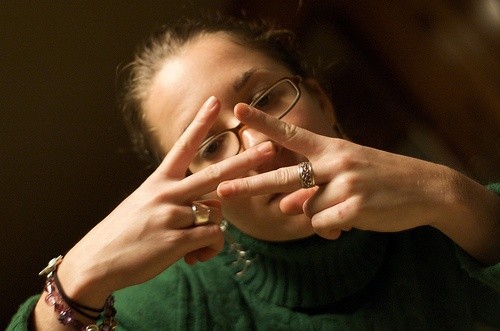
[38,254,119,331]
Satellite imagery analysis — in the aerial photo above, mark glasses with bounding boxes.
[186,74,302,174]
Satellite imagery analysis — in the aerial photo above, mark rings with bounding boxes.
[298,161,316,189]
[190,202,211,226]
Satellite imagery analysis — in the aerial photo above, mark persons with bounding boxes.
[4,13,500,331]
